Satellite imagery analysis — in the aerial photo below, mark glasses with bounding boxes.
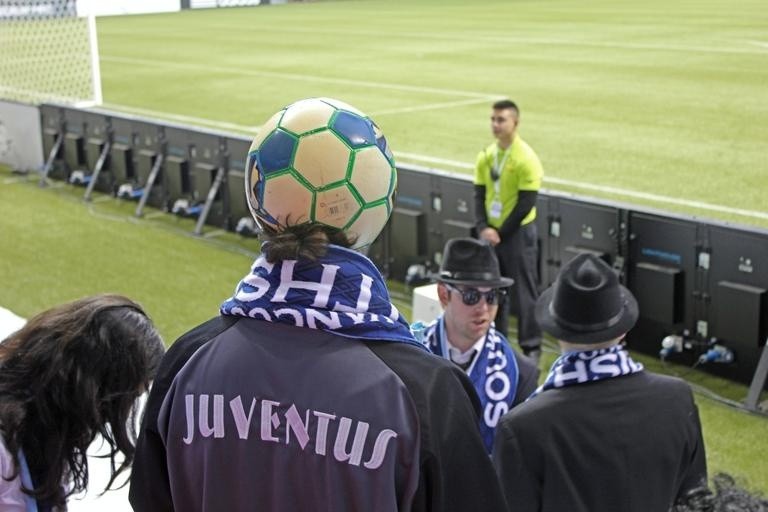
[451,283,507,305]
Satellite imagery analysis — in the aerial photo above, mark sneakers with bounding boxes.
[523,346,541,368]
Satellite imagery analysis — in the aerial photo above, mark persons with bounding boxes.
[405,237,542,458]
[471,100,543,358]
[491,251,706,512]
[667,470,768,512]
[128,98,511,512]
[0,293,167,512]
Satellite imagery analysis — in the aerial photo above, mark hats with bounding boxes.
[534,252,640,344]
[424,237,515,288]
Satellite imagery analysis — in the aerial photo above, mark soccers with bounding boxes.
[243,99,399,258]
